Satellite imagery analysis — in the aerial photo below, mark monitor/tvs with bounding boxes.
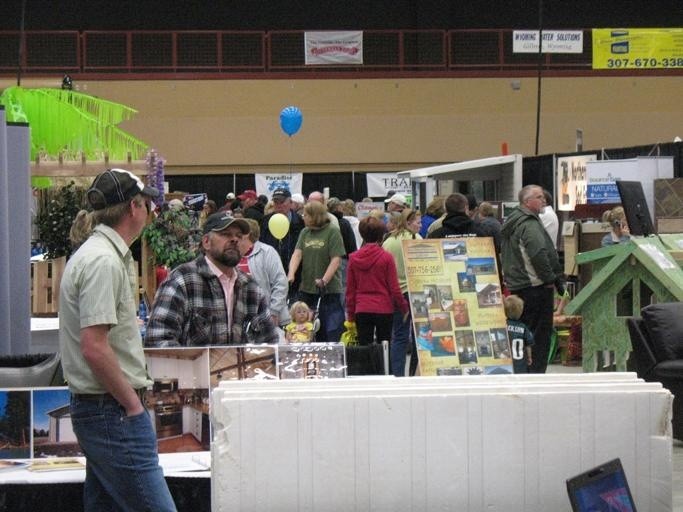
[617,180,655,235]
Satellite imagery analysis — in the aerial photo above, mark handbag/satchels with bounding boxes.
[316,302,345,342]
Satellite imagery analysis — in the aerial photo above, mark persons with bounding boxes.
[144,183,567,373]
[601,206,632,246]
[58,167,178,512]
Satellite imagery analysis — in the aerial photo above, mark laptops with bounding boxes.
[566,459,638,511]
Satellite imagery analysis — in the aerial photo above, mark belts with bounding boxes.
[71,387,147,406]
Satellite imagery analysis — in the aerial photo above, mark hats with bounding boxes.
[272,189,305,204]
[203,213,249,234]
[226,190,256,200]
[87,168,161,210]
[384,192,407,207]
[168,199,185,212]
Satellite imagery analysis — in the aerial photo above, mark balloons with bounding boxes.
[280,105,303,138]
